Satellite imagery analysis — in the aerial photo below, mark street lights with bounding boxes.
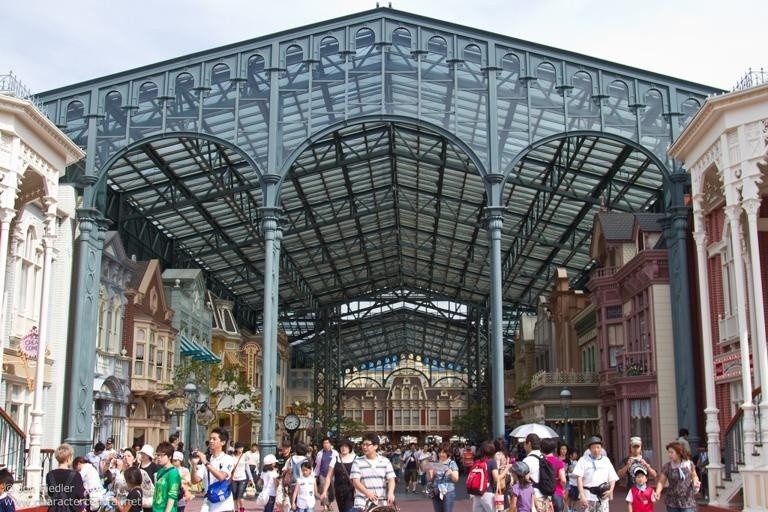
[558,385,572,447]
[183,380,200,468]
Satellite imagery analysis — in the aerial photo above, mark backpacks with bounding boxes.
[465,456,491,497]
[526,453,555,496]
[47,470,79,512]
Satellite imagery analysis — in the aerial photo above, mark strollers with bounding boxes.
[364,495,400,512]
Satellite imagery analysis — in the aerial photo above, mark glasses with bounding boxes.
[361,443,373,447]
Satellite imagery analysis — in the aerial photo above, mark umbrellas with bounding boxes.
[509,423,560,439]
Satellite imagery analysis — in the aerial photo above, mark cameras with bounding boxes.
[192,452,200,457]
[115,453,124,459]
[632,458,641,463]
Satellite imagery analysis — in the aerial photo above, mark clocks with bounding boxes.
[280,411,301,433]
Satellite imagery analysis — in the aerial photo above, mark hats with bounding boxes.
[511,461,531,477]
[633,465,648,475]
[264,454,280,465]
[630,437,642,447]
[586,436,603,450]
[336,438,353,453]
[394,448,403,455]
[172,451,184,461]
[138,444,156,460]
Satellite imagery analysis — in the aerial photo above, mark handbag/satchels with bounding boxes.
[276,478,291,512]
[427,481,445,498]
[207,477,232,504]
[283,455,292,486]
[229,469,236,486]
[141,492,155,508]
[255,473,272,507]
[246,481,257,497]
[590,482,610,501]
[393,462,400,470]
[494,476,505,511]
